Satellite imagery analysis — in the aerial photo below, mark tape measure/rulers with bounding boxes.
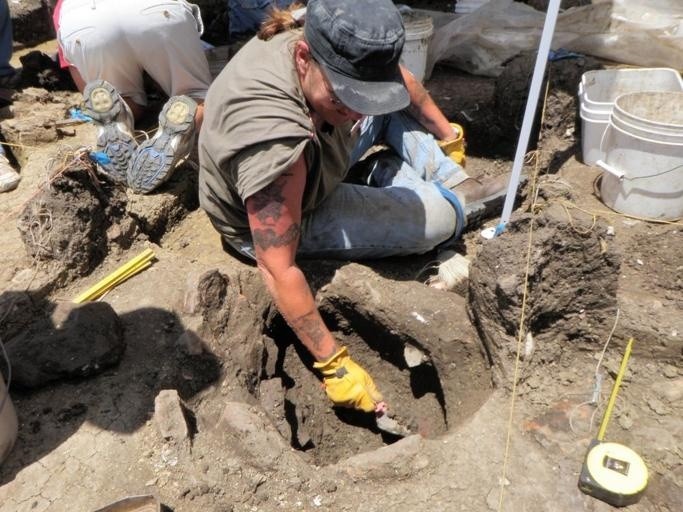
[577,337,649,509]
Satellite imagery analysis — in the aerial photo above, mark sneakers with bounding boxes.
[126,95,198,195]
[83,79,140,181]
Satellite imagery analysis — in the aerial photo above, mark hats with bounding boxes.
[304,0,410,116]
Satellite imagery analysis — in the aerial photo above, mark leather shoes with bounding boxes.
[450,172,529,234]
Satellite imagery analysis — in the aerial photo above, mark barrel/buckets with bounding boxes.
[577,67,683,168]
[398,10,434,85]
[596,91,683,222]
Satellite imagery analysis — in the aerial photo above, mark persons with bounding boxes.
[197,0,532,418]
[0,0,23,195]
[50,0,213,196]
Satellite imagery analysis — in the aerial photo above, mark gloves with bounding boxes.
[434,123,466,168]
[312,346,383,414]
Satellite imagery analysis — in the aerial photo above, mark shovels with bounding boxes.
[375,401,410,436]
